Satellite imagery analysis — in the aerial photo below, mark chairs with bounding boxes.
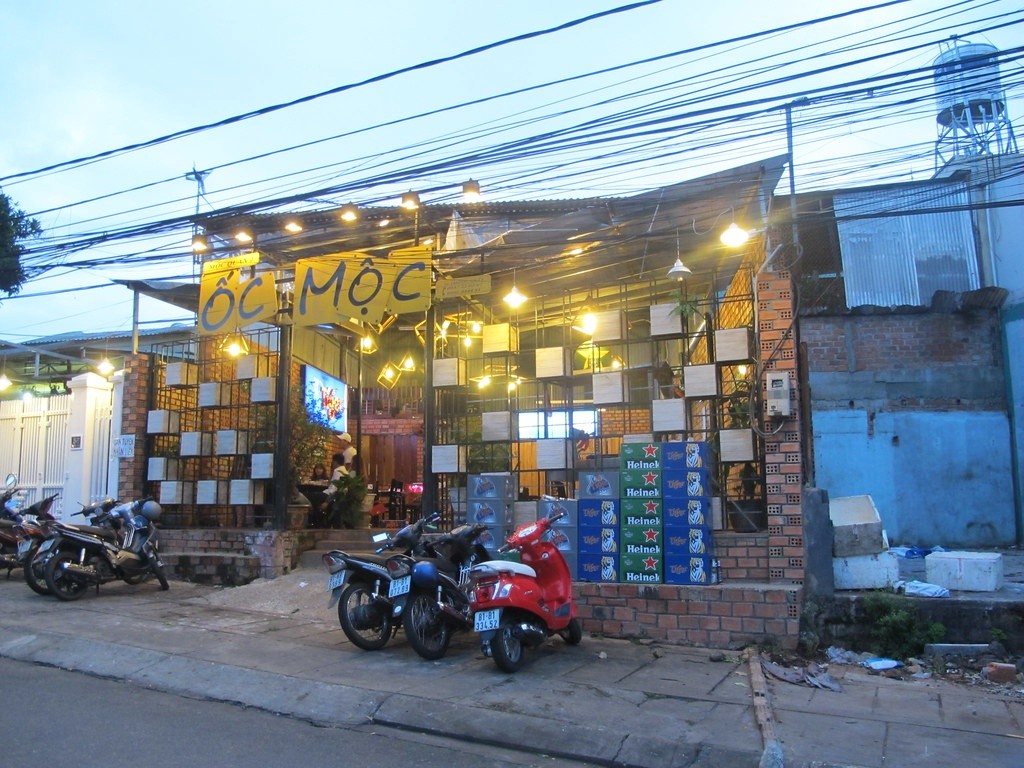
[382,478,404,520]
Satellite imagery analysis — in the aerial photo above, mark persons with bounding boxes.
[317,454,366,523]
[309,462,329,481]
[335,432,358,472]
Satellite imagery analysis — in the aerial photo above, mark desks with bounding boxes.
[296,484,329,528]
[367,488,406,520]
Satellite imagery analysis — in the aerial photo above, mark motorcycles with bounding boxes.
[323,512,442,652]
[39,496,170,604]
[13,498,119,595]
[0,486,21,516]
[462,511,583,675]
[385,518,496,661]
[0,493,61,570]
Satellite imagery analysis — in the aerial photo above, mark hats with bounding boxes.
[336,432,352,444]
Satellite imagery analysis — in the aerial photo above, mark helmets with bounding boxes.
[142,501,162,520]
[411,561,439,589]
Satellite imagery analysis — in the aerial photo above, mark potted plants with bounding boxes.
[702,380,758,531]
[326,472,377,530]
[726,462,766,533]
[447,416,514,523]
[240,371,336,530]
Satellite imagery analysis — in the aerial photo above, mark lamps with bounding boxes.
[398,330,416,372]
[377,327,402,391]
[667,228,692,283]
[341,204,358,221]
[353,327,381,354]
[401,192,417,208]
[191,233,207,251]
[502,268,528,307]
[97,339,115,374]
[571,292,601,335]
[217,327,249,358]
[462,180,480,200]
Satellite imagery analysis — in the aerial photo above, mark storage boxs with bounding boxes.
[663,553,717,586]
[833,551,899,591]
[828,493,883,558]
[663,525,715,554]
[465,442,664,584]
[661,442,711,469]
[925,551,1005,591]
[661,468,713,497]
[662,497,713,526]
